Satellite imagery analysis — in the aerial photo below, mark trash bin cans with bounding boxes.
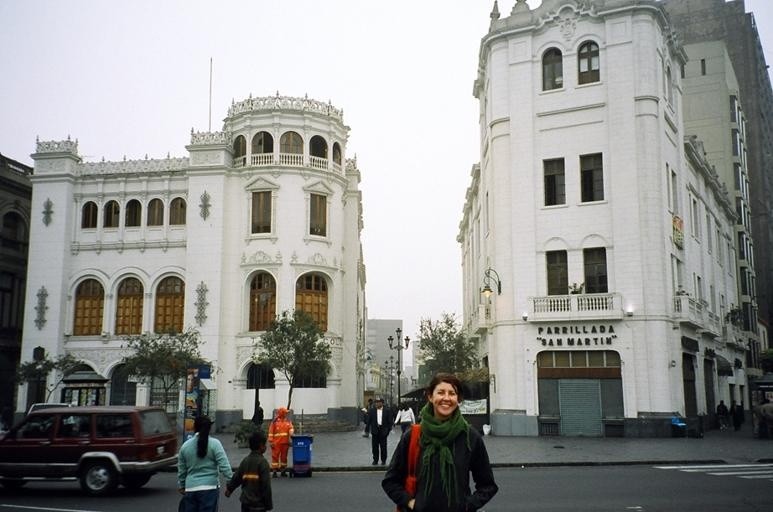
[289,435,313,478]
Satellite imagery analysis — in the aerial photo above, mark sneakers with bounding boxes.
[372,460,387,466]
[272,471,288,478]
[362,432,369,437]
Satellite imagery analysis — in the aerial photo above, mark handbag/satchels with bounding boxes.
[397,475,417,511]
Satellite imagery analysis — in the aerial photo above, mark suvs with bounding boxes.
[0,398,183,496]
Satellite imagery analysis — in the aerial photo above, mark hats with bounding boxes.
[194,414,215,426]
[277,406,290,418]
[373,397,385,403]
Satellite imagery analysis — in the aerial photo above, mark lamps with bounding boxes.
[627,310,634,317]
[478,266,502,299]
[521,312,529,323]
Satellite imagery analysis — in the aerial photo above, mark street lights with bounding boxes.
[383,326,409,408]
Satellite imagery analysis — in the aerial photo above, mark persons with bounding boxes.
[176,415,234,512]
[380,375,500,512]
[223,433,274,511]
[368,397,392,465]
[392,400,417,432]
[266,406,296,478]
[185,405,196,419]
[360,397,373,437]
[727,400,745,432]
[185,370,199,402]
[716,399,729,432]
